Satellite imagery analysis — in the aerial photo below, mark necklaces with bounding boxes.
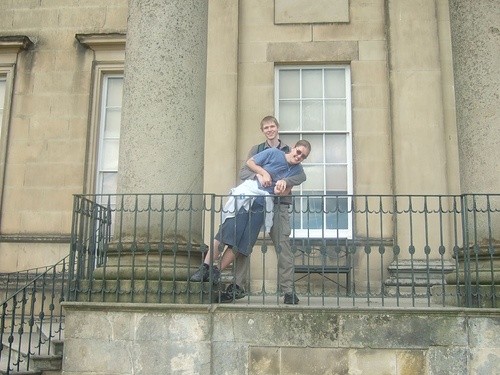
[286,160,295,172]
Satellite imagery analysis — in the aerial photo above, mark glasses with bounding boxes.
[295,146,307,159]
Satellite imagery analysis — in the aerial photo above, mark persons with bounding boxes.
[217,115,306,304]
[187,141,313,284]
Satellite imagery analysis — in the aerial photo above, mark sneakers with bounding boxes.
[284,294,299,304]
[189,262,210,282]
[206,269,220,284]
[226,284,246,299]
